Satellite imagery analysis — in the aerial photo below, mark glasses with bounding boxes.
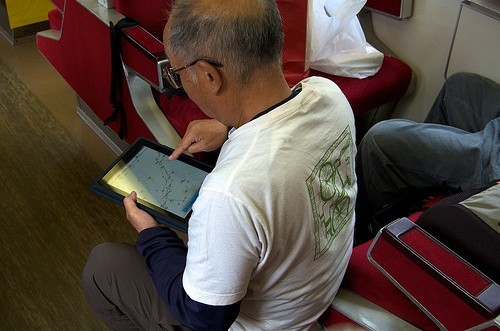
[163,58,224,89]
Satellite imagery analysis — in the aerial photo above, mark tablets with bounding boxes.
[89,137,214,234]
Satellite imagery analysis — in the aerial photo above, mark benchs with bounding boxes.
[309,181,500,331]
[35,0,417,158]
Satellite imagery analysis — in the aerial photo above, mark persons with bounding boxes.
[82,0,358,331]
[354,71,500,249]
